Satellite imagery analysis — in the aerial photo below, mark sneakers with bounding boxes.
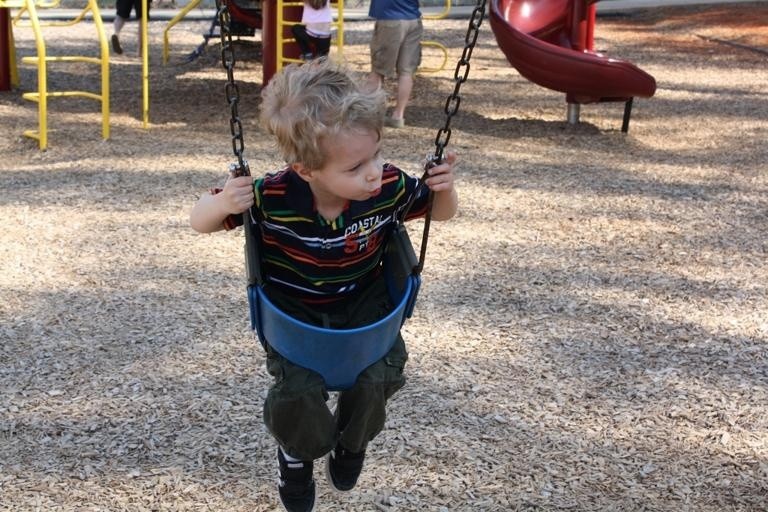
[111,34,123,55]
[275,444,317,512]
[384,115,406,128]
[299,53,312,62]
[325,403,367,493]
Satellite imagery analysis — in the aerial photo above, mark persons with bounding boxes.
[368,1,422,129]
[190,55,459,511]
[111,1,156,59]
[292,1,334,62]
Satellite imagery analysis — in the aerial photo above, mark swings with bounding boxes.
[214,1,486,389]
